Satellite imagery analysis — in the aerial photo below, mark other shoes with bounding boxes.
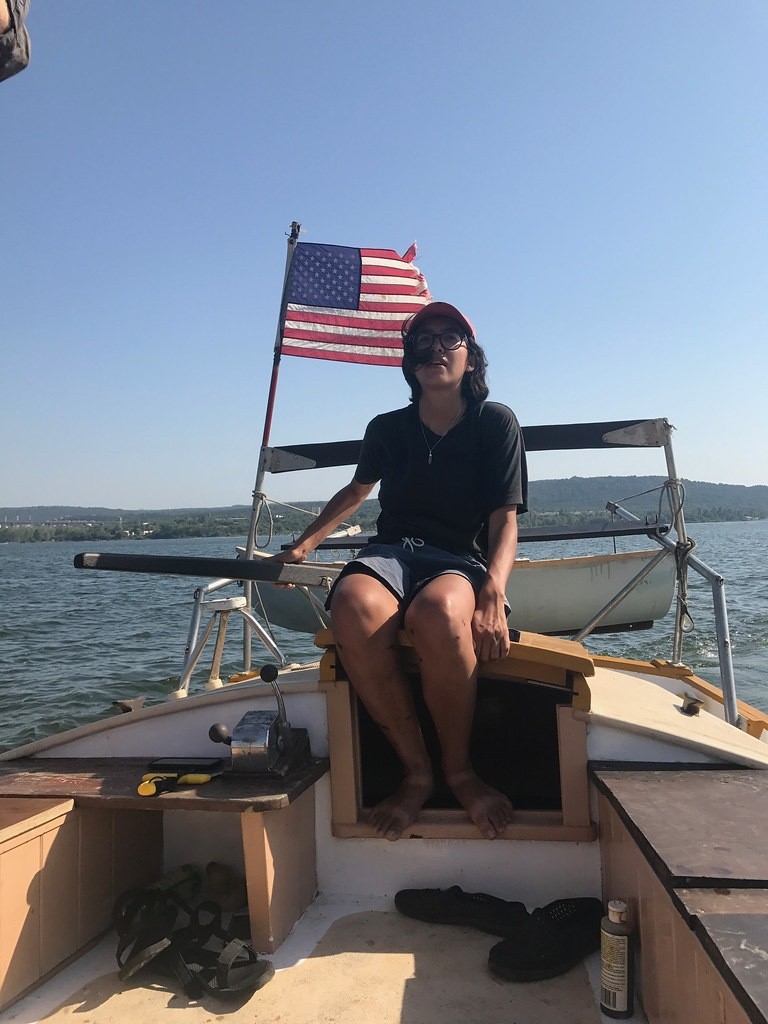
[488,897,607,981]
[394,885,530,939]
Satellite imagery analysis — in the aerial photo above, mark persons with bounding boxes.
[270,302,528,841]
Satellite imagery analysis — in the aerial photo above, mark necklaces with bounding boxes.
[418,405,463,466]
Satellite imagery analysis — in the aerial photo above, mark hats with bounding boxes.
[402,302,475,341]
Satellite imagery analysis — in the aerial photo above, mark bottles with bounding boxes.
[600,900,637,1020]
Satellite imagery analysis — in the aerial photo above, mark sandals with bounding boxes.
[112,888,275,1001]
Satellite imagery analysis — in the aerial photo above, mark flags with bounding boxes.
[278,238,427,367]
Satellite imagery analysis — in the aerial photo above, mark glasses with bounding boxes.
[411,331,469,352]
